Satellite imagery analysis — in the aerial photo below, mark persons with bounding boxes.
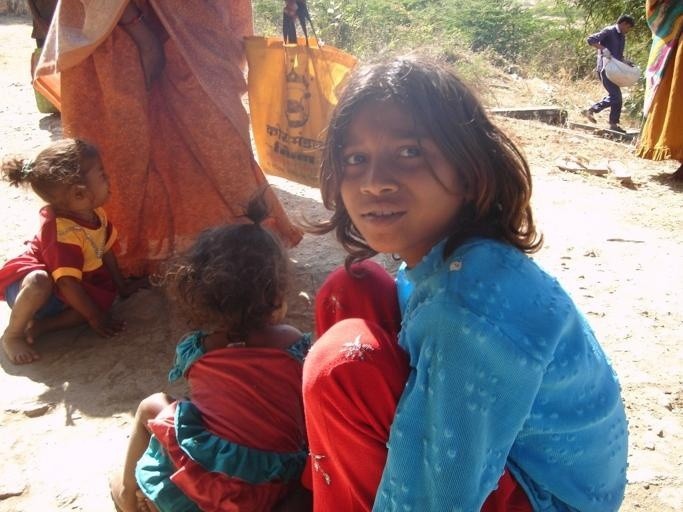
[26,1,58,115]
[581,14,637,133]
[0,136,142,368]
[293,53,627,511]
[33,0,302,293]
[111,219,321,510]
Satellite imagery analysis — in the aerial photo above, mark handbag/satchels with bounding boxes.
[242,0,360,190]
